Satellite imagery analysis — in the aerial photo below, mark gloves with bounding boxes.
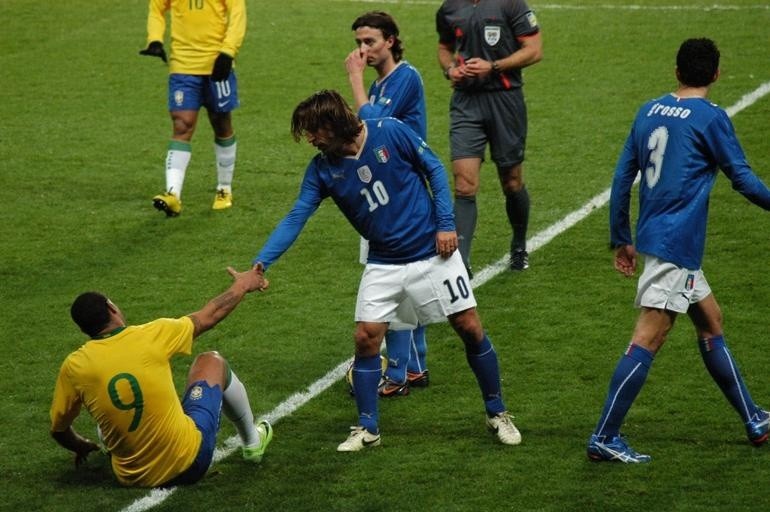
[211,52,233,82]
[139,41,167,63]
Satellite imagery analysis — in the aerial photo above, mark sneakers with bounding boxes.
[378,369,430,397]
[745,409,770,443]
[212,188,232,210]
[336,426,381,452]
[587,433,652,464]
[152,191,182,216]
[485,411,522,446]
[242,420,273,462]
[510,248,529,270]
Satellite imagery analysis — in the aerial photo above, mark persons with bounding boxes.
[342,10,431,396]
[252,89,525,453]
[48,267,275,487]
[434,1,543,279]
[139,2,246,216]
[585,38,770,462]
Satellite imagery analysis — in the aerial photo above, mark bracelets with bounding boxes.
[444,65,452,81]
[491,60,498,73]
